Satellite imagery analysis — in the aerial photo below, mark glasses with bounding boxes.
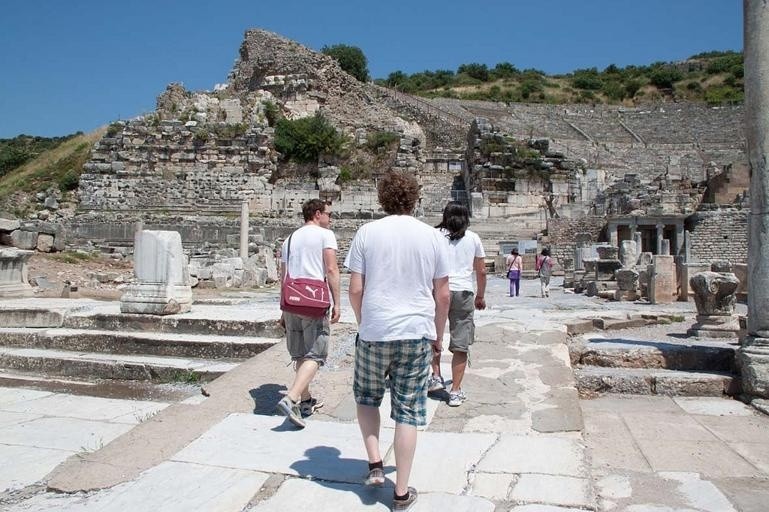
[322,211,333,217]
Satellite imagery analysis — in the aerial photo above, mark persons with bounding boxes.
[536,247,553,298]
[428,201,486,407]
[505,247,522,297]
[277,198,340,429]
[343,171,450,512]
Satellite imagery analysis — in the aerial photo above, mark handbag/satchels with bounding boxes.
[280,274,330,320]
[507,270,510,277]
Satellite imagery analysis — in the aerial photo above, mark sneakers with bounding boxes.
[363,468,385,488]
[427,374,468,407]
[275,396,324,428]
[391,487,418,512]
